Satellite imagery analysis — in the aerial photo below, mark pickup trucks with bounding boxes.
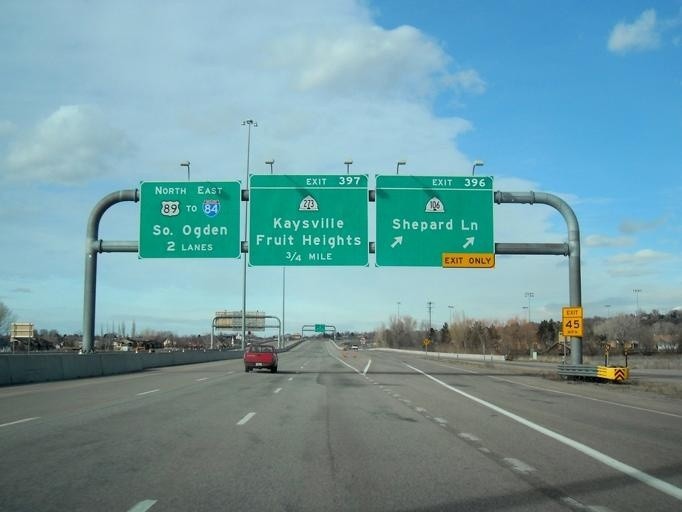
[243,344,279,373]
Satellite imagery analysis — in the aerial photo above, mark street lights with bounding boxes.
[425,302,435,332]
[238,116,257,353]
[604,304,611,319]
[395,301,402,326]
[524,292,534,323]
[448,304,457,326]
[520,305,528,323]
[632,288,642,315]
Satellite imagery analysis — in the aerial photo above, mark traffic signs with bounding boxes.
[563,318,582,336]
[249,174,368,265]
[374,175,495,268]
[315,324,325,332]
[140,181,240,259]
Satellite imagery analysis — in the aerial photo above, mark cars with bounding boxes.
[352,344,359,351]
